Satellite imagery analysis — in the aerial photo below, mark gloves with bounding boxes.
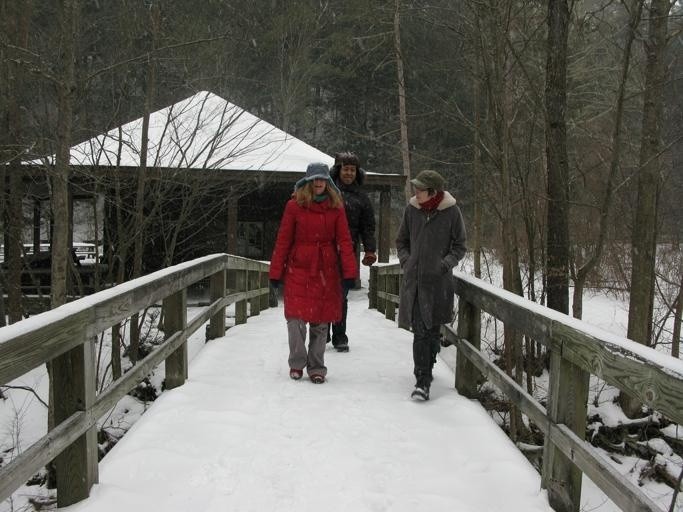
[342,279,355,289]
[269,279,279,288]
[361,251,376,266]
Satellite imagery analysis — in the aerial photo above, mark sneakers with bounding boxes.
[333,343,348,352]
[307,337,330,348]
[310,372,325,384]
[410,385,429,401]
[289,367,303,380]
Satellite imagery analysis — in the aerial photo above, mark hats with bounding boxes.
[328,152,365,186]
[407,170,444,193]
[294,162,340,197]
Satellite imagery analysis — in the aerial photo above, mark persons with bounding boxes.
[325,149,376,353]
[395,168,467,403]
[268,162,357,384]
